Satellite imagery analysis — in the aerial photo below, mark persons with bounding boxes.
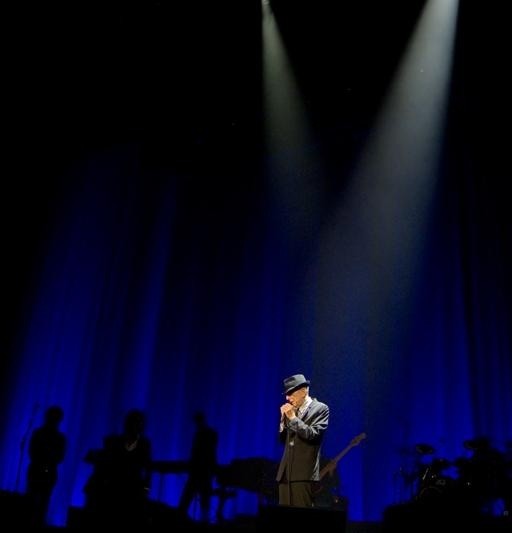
[25,405,67,521]
[179,407,218,524]
[275,374,329,509]
[103,409,153,503]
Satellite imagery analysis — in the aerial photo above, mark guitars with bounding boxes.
[314,432,367,493]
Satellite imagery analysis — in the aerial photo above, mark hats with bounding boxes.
[282,374,311,396]
